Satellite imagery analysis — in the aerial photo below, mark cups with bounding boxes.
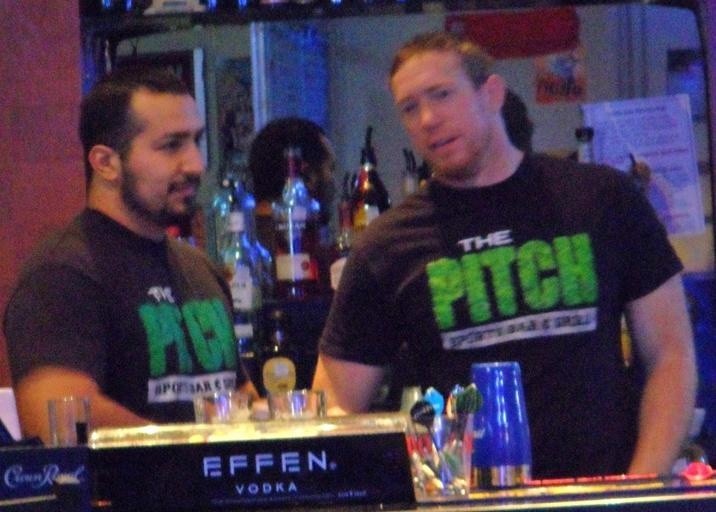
[193,390,248,428]
[46,396,95,446]
[264,388,328,423]
[404,413,476,504]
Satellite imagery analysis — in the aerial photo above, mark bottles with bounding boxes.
[575,127,594,166]
[470,361,533,487]
[207,141,423,353]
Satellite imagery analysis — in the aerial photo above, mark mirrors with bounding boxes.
[81,5,710,300]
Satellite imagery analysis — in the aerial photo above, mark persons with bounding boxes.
[499,86,652,199]
[2,58,263,449]
[241,114,367,395]
[317,30,700,482]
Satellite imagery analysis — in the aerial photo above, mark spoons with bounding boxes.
[404,381,483,494]
